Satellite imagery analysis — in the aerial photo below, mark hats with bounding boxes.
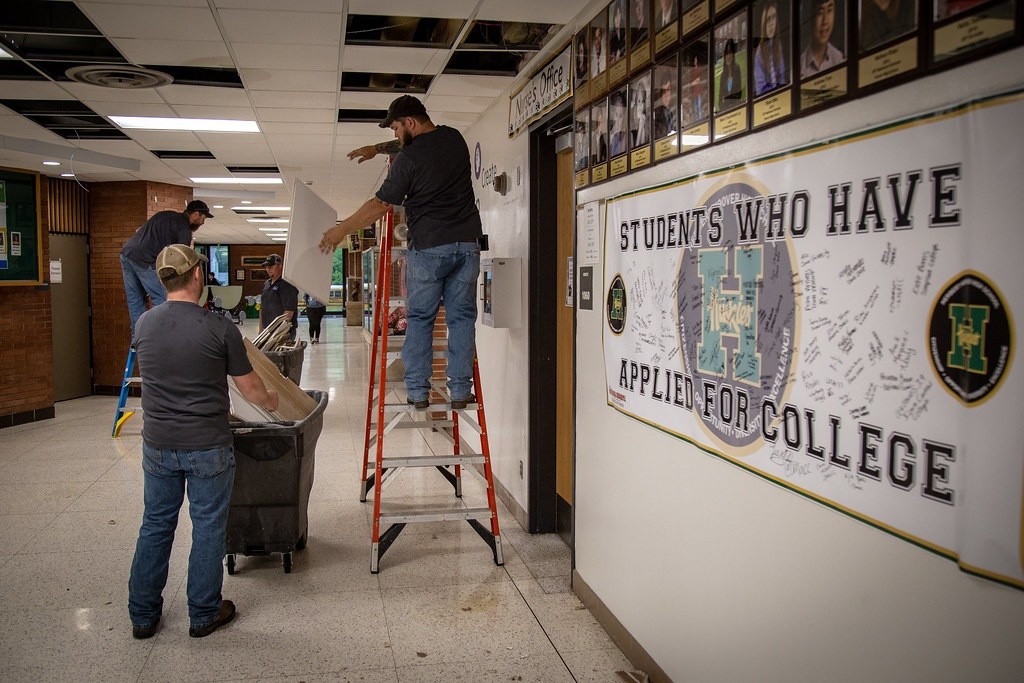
[261,253,284,267]
[378,95,427,128]
[155,243,209,280]
[187,199,215,218]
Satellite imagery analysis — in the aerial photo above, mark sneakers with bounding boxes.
[188,598,237,637]
[407,396,430,408]
[450,393,476,409]
[310,336,320,344]
[133,617,159,638]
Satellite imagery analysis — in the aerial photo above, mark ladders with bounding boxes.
[356,199,511,576]
[113,293,155,438]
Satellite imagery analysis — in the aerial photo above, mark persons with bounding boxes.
[257,253,299,341]
[655,0,676,32]
[654,71,677,140]
[575,120,589,171]
[717,39,743,112]
[630,0,649,50]
[574,35,588,88]
[303,292,327,345]
[799,1,845,80]
[13,234,19,244]
[682,53,703,121]
[753,1,787,98]
[591,107,608,164]
[630,81,649,148]
[317,94,484,410]
[590,23,606,79]
[126,243,280,640]
[861,0,916,50]
[609,0,625,65]
[609,91,628,157]
[208,272,221,287]
[119,199,215,351]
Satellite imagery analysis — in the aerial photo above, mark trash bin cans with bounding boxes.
[225,389,330,576]
[249,340,308,389]
